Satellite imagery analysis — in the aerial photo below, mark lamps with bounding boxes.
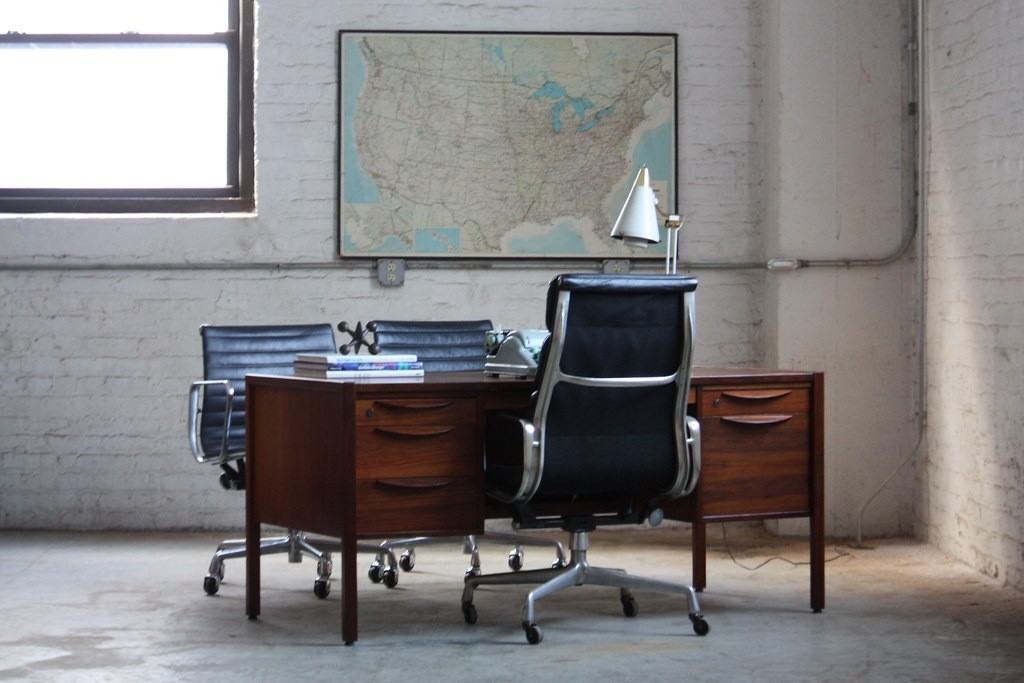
[609,162,683,275]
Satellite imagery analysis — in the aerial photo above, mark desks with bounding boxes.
[245,366,825,646]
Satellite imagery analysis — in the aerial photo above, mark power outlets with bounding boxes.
[378,259,405,286]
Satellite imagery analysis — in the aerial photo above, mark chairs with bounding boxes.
[187,320,399,599]
[368,319,567,582]
[462,273,709,645]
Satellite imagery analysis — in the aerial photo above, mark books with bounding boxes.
[293,355,425,379]
